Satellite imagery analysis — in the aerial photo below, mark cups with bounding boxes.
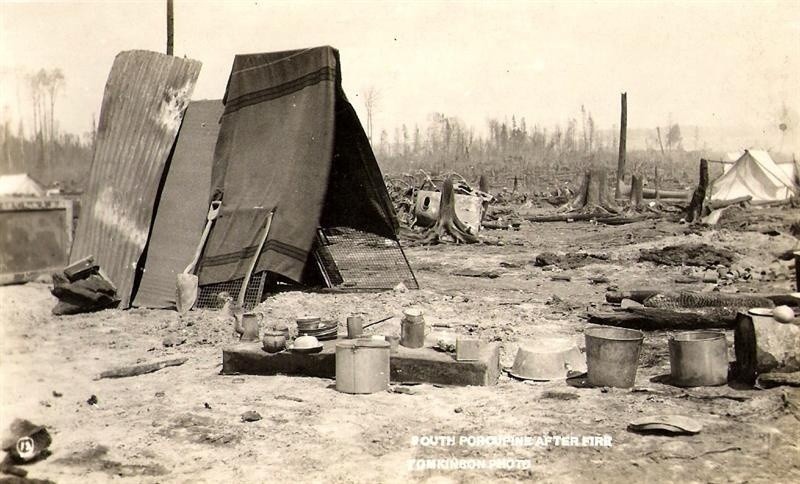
[347,316,362,339]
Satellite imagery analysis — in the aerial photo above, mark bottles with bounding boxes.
[400,308,432,349]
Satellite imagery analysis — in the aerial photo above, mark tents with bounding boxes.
[705,145,799,209]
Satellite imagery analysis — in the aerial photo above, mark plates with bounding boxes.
[298,321,338,340]
[749,307,800,318]
[288,343,324,350]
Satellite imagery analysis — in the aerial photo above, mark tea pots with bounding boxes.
[233,312,263,342]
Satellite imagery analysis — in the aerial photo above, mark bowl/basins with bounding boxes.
[510,338,588,381]
[295,317,321,331]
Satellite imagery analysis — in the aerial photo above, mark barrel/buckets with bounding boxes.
[668,331,730,387]
[585,325,645,389]
[335,338,391,394]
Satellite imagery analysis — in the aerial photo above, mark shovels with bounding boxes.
[174,200,221,312]
